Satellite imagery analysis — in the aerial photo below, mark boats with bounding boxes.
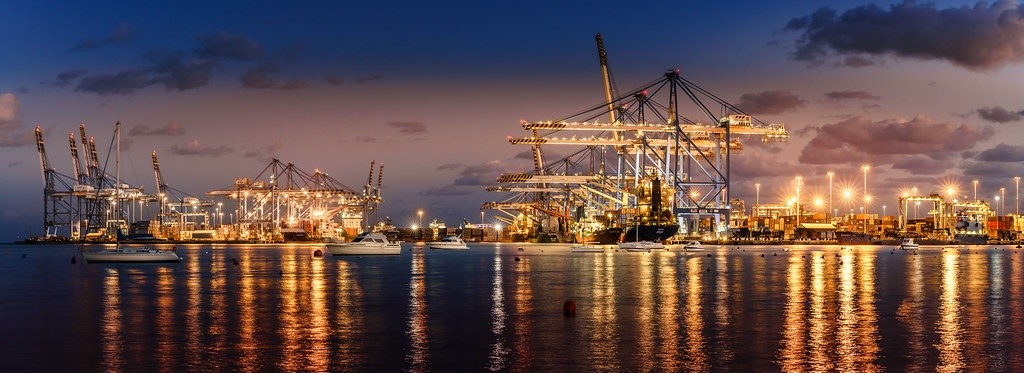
[900,238,919,250]
[324,231,402,254]
[424,235,471,250]
[571,244,606,253]
[683,240,705,252]
[619,242,664,252]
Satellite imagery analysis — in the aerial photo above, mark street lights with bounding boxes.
[791,165,1021,241]
[481,212,485,223]
[418,210,423,227]
[721,187,726,202]
[493,224,502,242]
[689,189,699,208]
[754,182,762,218]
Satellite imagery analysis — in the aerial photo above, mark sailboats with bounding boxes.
[78,122,185,264]
